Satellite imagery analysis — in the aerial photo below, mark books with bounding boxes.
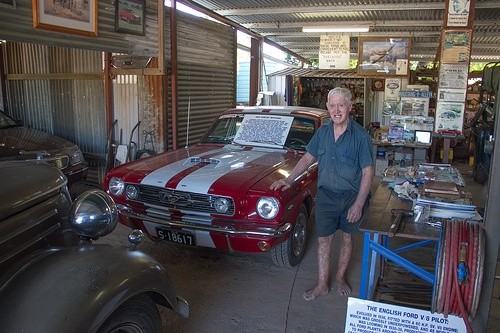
[424,179,458,195]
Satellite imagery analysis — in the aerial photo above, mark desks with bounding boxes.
[373,139,430,177]
[358,181,440,301]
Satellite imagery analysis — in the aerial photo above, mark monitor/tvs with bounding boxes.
[414,130,432,146]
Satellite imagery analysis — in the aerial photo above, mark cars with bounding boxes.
[0,110,91,192]
[102,105,334,270]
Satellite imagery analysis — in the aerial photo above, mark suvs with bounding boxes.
[0,159,184,333]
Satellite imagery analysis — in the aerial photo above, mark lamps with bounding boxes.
[302,26,369,33]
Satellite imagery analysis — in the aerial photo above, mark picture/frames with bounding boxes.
[357,37,411,77]
[114,0,146,37]
[31,0,98,38]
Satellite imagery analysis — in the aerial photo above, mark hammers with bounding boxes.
[388,208,414,237]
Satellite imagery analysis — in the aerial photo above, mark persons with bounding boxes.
[270,87,374,302]
